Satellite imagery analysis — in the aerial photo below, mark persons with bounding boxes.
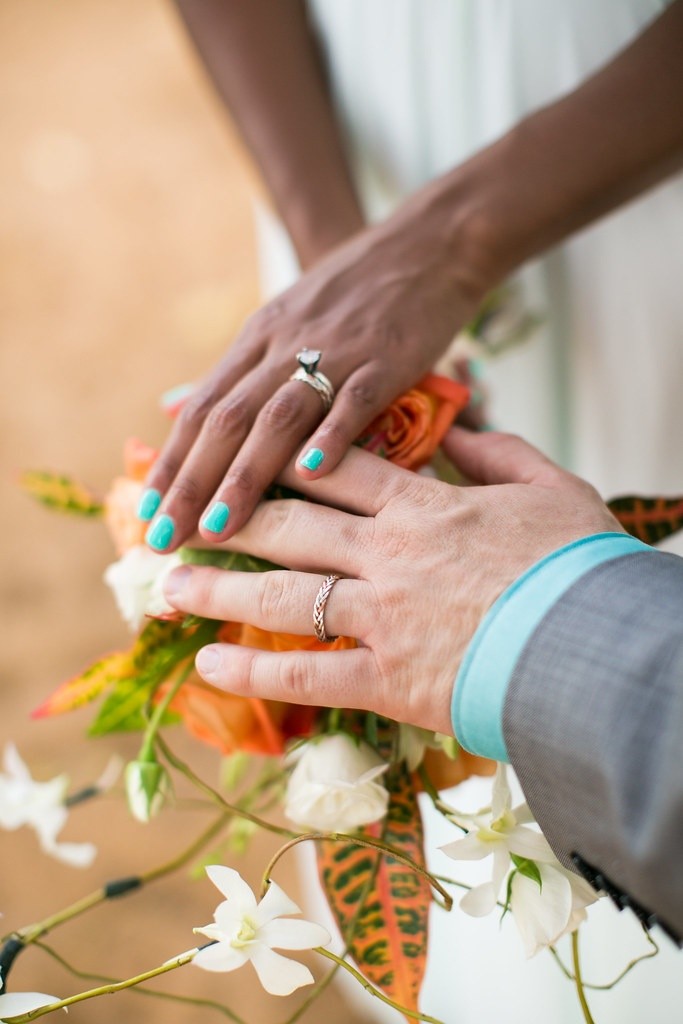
[128,0,683,1024]
[139,376,683,946]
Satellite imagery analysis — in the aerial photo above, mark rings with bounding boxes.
[313,575,344,642]
[288,347,335,411]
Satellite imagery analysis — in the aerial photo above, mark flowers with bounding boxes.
[0,275,682,1024]
